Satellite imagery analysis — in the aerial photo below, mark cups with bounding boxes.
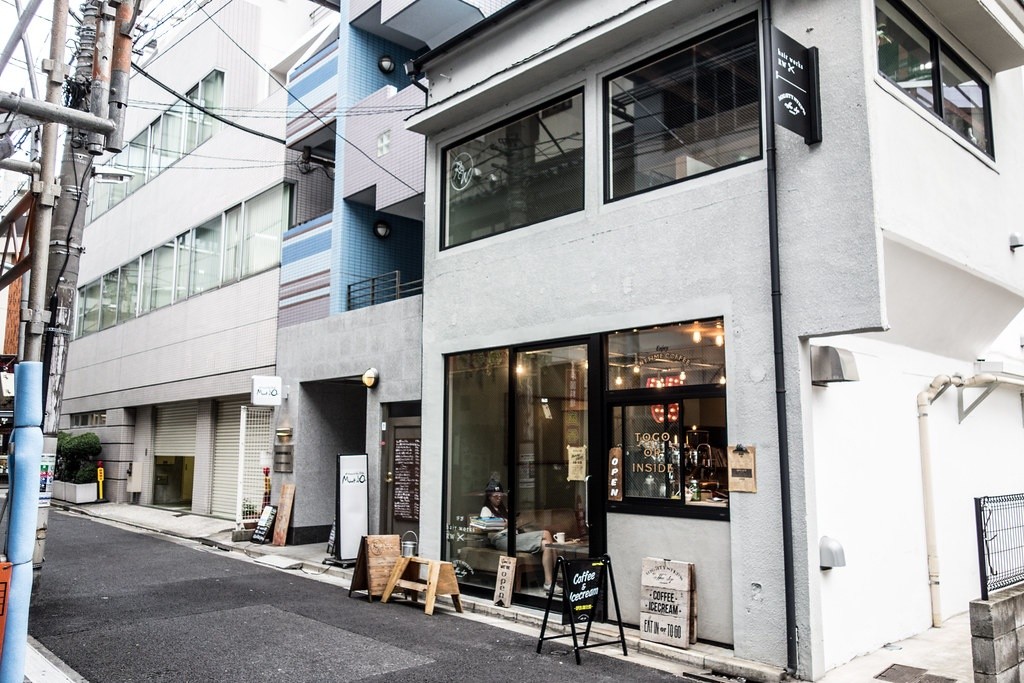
[553,532,566,544]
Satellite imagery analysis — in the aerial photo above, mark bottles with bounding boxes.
[642,475,658,498]
[690,480,700,500]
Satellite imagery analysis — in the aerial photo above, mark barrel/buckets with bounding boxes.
[400,530,419,558]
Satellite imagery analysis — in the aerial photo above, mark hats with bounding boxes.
[484,479,503,493]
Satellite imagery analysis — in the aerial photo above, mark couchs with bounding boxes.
[460,509,591,589]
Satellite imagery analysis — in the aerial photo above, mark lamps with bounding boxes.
[719,367,727,385]
[615,367,624,385]
[693,322,702,344]
[634,366,641,374]
[680,364,686,382]
[715,323,724,347]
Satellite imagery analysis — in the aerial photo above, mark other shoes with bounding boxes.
[543,582,563,593]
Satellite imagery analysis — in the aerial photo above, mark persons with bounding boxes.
[479,478,564,595]
